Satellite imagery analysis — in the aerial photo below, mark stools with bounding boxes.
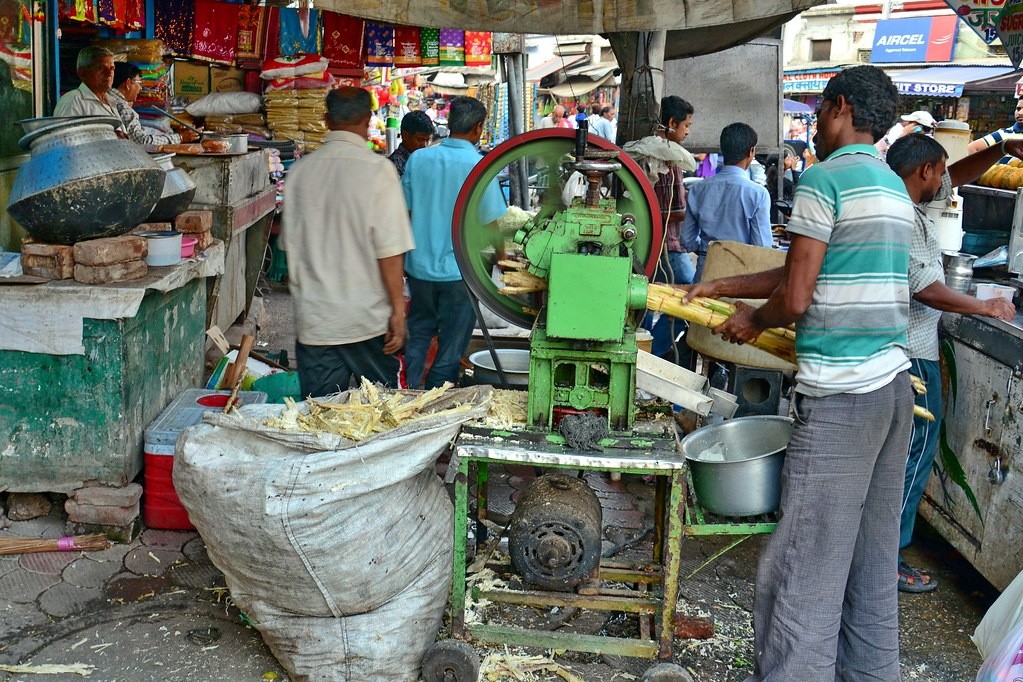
[726,361,784,419]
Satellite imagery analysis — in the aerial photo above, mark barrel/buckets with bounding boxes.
[252,372,301,402]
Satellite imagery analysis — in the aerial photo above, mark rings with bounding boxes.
[397,339,403,342]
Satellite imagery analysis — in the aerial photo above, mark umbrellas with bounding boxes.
[780,98,814,115]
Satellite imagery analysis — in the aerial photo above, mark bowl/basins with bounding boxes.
[974,283,1016,301]
[468,349,529,385]
[680,415,796,516]
[133,230,198,268]
[16,114,109,134]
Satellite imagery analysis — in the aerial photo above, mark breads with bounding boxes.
[161,138,230,153]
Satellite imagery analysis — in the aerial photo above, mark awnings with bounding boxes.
[884,65,1023,98]
[525,54,585,85]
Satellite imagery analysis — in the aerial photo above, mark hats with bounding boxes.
[900,110,936,129]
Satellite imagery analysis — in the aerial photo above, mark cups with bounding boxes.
[942,252,979,293]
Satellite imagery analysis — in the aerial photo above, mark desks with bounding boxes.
[0,235,222,492]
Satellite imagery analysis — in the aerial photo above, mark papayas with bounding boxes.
[976,158,1023,191]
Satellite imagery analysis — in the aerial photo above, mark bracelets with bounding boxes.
[178,133,184,141]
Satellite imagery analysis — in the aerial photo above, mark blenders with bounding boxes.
[925,120,972,251]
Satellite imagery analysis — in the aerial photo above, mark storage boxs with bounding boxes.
[204,325,289,388]
[142,388,268,530]
[271,239,289,282]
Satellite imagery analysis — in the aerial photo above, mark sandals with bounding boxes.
[898,561,940,594]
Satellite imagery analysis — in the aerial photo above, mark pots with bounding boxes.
[150,104,250,154]
[773,200,792,218]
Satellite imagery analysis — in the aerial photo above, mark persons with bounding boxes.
[399,95,506,391]
[640,94,696,358]
[52,45,127,133]
[967,90,1023,167]
[875,111,935,159]
[651,64,916,682]
[679,122,773,390]
[691,147,801,224]
[888,132,1016,594]
[275,86,416,400]
[106,60,205,154]
[535,101,617,143]
[788,114,817,157]
[386,108,436,176]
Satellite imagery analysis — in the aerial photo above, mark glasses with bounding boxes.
[132,80,143,87]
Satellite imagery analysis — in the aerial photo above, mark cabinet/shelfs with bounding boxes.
[916,304,1023,595]
